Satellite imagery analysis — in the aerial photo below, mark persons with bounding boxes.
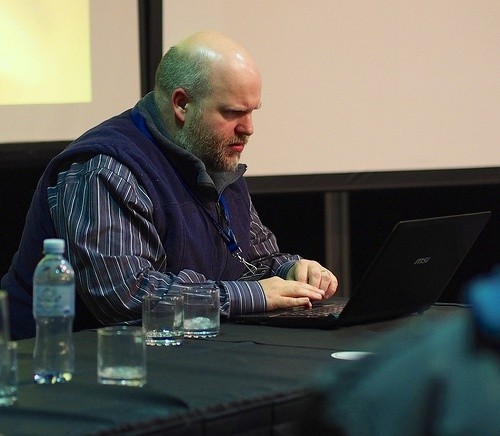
[4,28,339,340]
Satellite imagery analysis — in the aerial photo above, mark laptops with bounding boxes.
[232,210,492,328]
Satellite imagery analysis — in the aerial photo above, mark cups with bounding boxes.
[141,294,185,346]
[173,286,221,339]
[96,326,147,387]
[0,341,19,406]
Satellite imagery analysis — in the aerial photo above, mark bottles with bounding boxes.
[31,238,77,384]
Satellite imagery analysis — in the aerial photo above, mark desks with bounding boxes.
[0,303,474,436]
